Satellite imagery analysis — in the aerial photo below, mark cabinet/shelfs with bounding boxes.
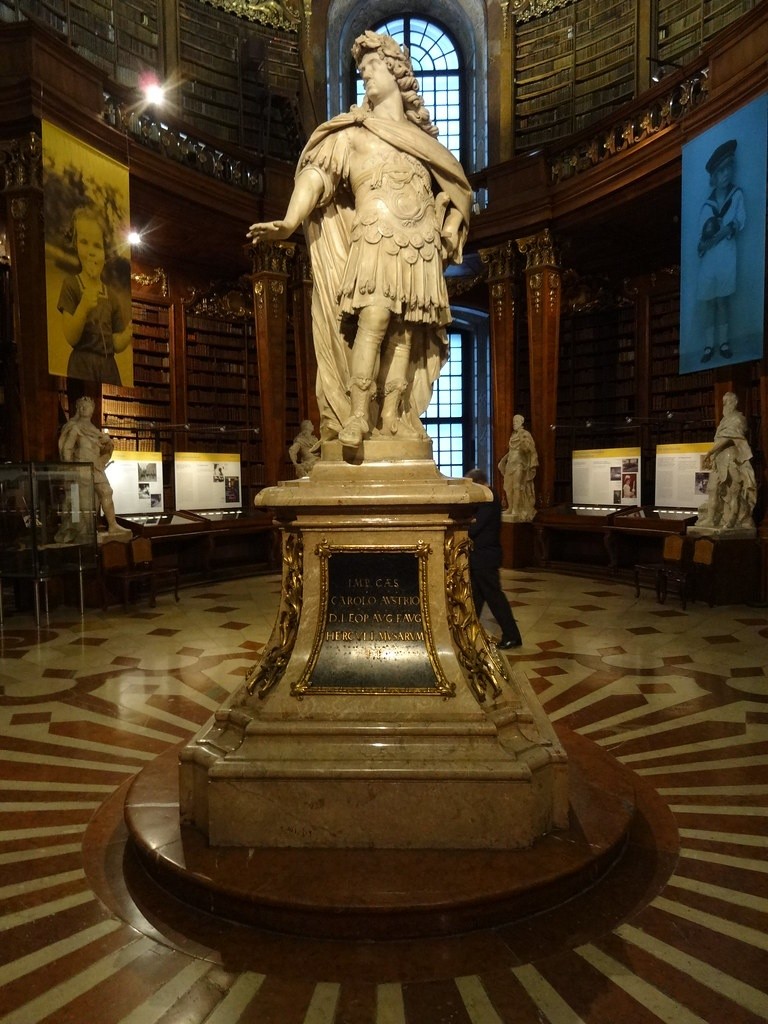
[500,0,768,164]
[512,264,768,516]
[51,273,316,510]
[0,0,304,163]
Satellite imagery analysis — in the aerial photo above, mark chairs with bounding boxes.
[632,533,689,603]
[661,536,719,612]
[127,536,182,609]
[97,540,156,614]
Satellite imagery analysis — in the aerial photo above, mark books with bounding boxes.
[515,0,768,147]
[0,0,305,164]
[512,262,768,505]
[60,301,300,507]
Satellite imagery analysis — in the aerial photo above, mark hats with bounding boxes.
[624,475,630,482]
[705,139,737,177]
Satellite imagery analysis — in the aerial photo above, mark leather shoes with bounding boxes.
[495,639,522,649]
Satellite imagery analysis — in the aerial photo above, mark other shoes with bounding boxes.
[700,345,715,362]
[719,342,732,359]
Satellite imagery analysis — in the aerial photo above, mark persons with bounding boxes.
[248,31,472,446]
[503,415,539,522]
[58,208,132,388]
[695,392,756,529]
[289,421,321,479]
[58,397,130,534]
[466,470,522,648]
[697,140,745,363]
[623,475,635,498]
